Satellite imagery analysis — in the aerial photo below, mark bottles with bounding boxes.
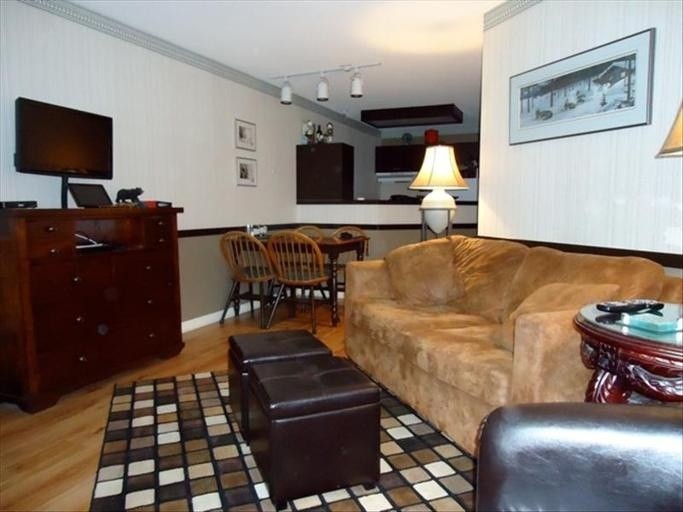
[246,223,267,238]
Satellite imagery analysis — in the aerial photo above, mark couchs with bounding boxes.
[341,235,682,466]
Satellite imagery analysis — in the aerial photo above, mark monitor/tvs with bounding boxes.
[14,97,112,178]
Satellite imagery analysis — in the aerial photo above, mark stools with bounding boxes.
[243,353,382,509]
[225,330,334,445]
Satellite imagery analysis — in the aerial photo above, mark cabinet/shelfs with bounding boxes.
[0,207,187,414]
[374,143,478,181]
[296,143,353,205]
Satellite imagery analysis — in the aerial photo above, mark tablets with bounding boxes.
[69,183,112,209]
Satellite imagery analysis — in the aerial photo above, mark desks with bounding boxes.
[567,296,683,407]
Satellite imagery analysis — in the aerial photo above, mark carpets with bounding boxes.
[87,351,477,512]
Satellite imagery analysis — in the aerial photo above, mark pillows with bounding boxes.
[379,235,464,307]
[492,279,621,360]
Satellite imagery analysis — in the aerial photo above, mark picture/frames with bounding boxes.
[507,25,656,147]
[235,156,257,187]
[233,117,256,151]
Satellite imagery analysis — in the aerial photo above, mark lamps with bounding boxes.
[269,62,383,106]
[360,103,463,129]
[653,101,683,160]
[407,141,470,235]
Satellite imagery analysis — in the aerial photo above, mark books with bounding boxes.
[142,200,172,208]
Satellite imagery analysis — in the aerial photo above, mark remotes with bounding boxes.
[599,300,664,313]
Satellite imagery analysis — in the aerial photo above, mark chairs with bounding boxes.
[217,223,372,335]
[474,397,682,511]
